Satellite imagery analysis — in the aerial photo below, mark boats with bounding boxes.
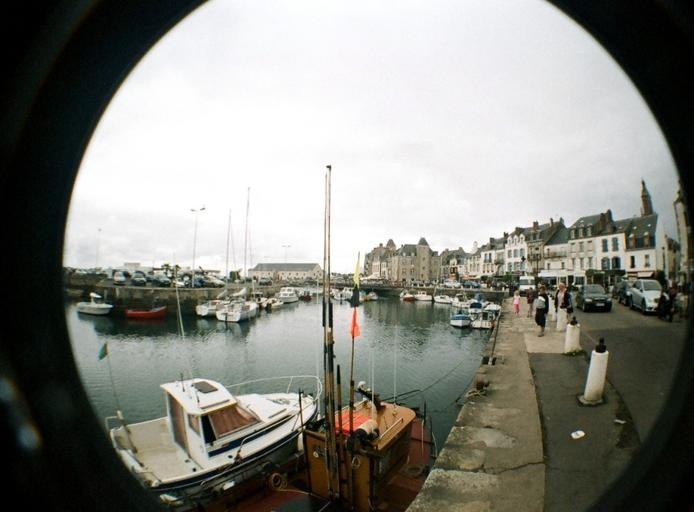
[124,303,169,321]
[95,270,322,494]
[167,156,437,512]
[398,289,454,305]
[75,293,115,316]
[470,308,494,330]
[448,303,471,329]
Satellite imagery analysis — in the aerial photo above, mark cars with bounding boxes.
[261,284,378,311]
[107,262,315,288]
[440,274,670,315]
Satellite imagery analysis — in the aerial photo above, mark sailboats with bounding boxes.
[215,185,261,324]
[195,213,232,320]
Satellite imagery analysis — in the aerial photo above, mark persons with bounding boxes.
[525,287,534,318]
[511,290,521,314]
[658,285,673,322]
[534,285,549,337]
[667,283,678,299]
[554,283,572,332]
[565,285,574,314]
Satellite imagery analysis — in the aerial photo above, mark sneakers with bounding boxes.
[530,314,533,317]
[538,334,544,337]
[527,315,529,317]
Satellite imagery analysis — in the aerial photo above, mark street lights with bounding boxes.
[94,227,102,268]
[534,246,540,278]
[188,206,208,288]
[281,244,292,262]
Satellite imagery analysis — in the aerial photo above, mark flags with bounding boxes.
[98,343,107,363]
[353,255,361,289]
[349,310,362,338]
